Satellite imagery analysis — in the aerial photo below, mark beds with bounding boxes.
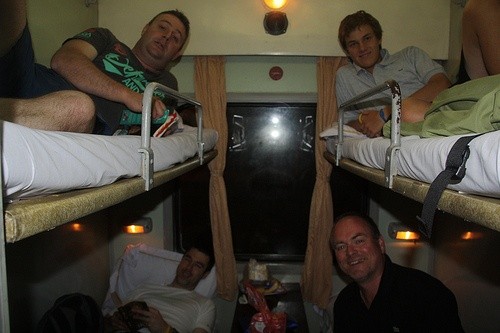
[303,81,500,333]
[0,80,239,333]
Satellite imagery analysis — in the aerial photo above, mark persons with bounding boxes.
[330,214,462,333]
[0,0,191,138]
[36,243,216,333]
[336,0,500,139]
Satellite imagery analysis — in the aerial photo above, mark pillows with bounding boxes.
[102,244,217,310]
[319,122,363,136]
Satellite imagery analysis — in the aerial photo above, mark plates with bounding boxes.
[239,281,290,295]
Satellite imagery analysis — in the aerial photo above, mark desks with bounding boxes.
[231,282,311,333]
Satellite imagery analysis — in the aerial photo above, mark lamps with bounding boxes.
[262,0,289,11]
[120,217,152,234]
[388,222,420,240]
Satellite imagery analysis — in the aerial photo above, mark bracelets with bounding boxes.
[380,109,386,122]
[358,113,362,123]
